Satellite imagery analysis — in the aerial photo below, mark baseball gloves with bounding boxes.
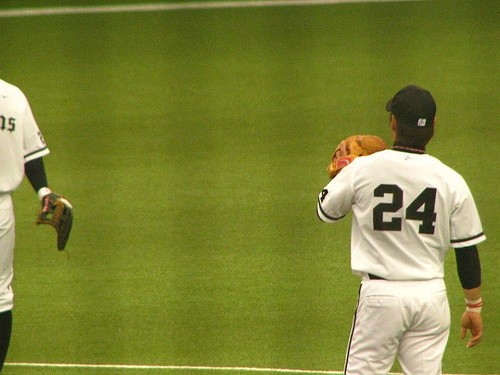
[36,191,72,254]
[326,129,386,179]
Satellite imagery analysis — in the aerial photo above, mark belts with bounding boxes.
[368,273,383,279]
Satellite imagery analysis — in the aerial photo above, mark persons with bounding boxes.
[0,78,73,375]
[317,85,484,375]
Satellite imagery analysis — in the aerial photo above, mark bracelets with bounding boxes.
[465,298,482,313]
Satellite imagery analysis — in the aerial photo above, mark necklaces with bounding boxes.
[391,145,425,155]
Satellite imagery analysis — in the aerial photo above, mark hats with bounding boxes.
[386,86,436,128]
